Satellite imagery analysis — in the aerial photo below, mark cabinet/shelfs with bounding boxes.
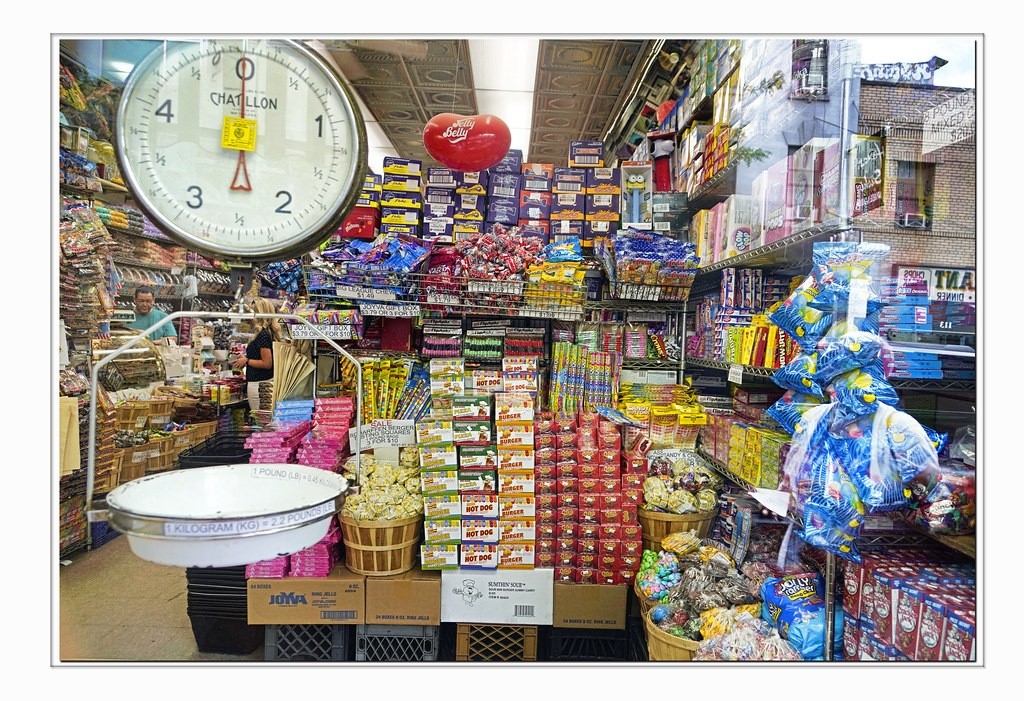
[600,38,978,663]
[59,52,249,557]
[215,253,689,663]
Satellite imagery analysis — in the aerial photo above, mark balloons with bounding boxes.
[421,113,511,173]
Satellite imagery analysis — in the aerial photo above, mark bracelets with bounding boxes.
[246,360,249,366]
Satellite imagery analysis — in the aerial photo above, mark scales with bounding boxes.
[83,40,369,569]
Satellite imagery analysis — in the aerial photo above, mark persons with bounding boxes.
[123,287,177,345]
[230,297,282,425]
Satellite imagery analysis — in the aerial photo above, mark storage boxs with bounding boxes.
[248,38,975,662]
